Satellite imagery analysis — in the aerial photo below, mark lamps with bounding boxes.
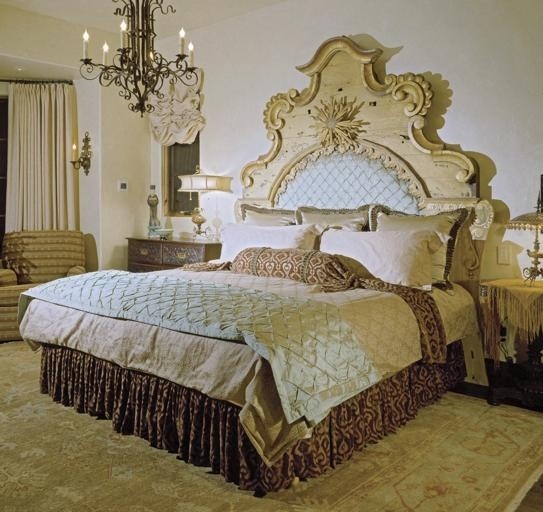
[79,0,198,117]
[70,130,94,176]
[178,166,234,241]
[506,173,543,285]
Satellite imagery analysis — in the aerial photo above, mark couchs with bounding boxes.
[0,230,87,344]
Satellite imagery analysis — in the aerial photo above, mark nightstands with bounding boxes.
[475,280,543,410]
[123,237,222,274]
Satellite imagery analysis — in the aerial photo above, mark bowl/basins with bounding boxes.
[154,229,173,237]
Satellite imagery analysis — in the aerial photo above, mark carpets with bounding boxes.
[0,338,542,510]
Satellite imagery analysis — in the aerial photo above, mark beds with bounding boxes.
[20,37,495,496]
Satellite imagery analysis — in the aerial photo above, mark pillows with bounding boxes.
[240,203,296,225]
[228,245,343,288]
[221,221,317,250]
[367,206,472,298]
[293,204,371,231]
[318,228,434,291]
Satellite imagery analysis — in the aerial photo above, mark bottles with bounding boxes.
[146,185,161,231]
